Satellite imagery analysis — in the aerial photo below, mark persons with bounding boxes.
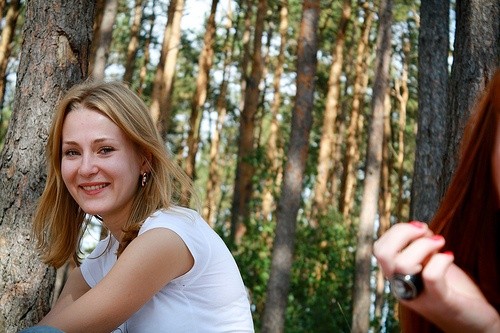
[372,68,500,333]
[16,81,255,333]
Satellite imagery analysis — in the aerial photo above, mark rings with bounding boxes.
[392,271,425,301]
